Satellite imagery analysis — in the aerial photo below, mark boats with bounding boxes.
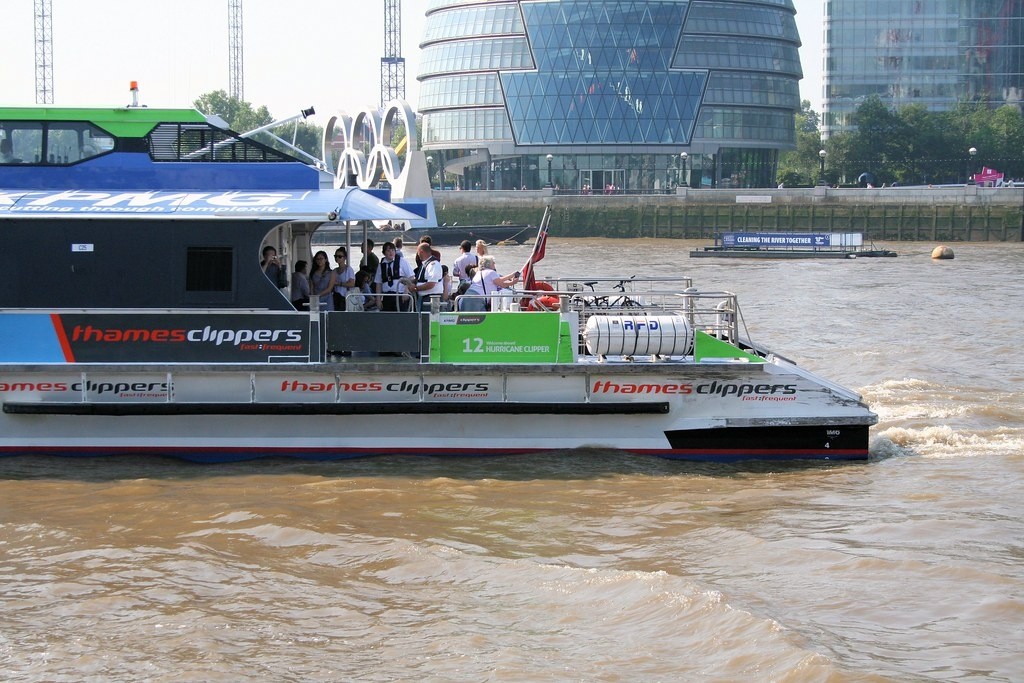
[0,80,880,463]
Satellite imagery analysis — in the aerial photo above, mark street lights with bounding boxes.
[427,156,433,182]
[967,147,977,185]
[544,153,554,187]
[817,149,826,186]
[678,151,689,187]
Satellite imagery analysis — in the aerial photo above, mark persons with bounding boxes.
[260,247,288,290]
[881,182,886,188]
[555,184,560,194]
[891,180,898,186]
[605,184,620,195]
[522,184,527,190]
[513,186,517,190]
[1000,179,1014,188]
[582,184,591,195]
[832,184,840,188]
[867,183,873,188]
[457,185,461,190]
[778,183,783,188]
[292,236,520,357]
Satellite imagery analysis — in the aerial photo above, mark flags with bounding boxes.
[524,223,548,284]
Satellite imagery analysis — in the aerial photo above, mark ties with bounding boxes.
[387,261,393,287]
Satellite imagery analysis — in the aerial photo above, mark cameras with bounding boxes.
[272,256,277,260]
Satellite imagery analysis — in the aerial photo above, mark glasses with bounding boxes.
[334,255,344,259]
[315,258,325,260]
[460,247,462,250]
[363,279,368,283]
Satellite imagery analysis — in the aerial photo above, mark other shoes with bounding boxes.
[326,349,351,357]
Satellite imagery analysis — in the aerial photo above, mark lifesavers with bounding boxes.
[520,282,559,311]
[528,297,560,312]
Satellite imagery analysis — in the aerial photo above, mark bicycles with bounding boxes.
[567,274,648,317]
